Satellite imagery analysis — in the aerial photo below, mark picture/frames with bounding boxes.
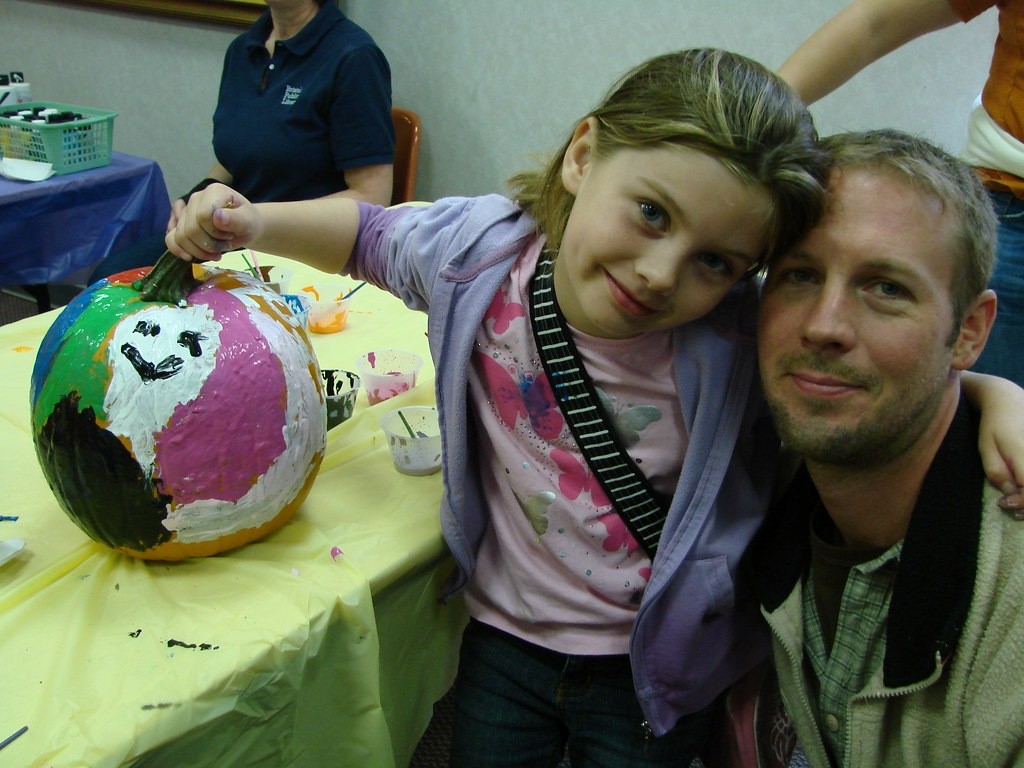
[86,0,267,26]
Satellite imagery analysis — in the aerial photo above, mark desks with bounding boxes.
[0,246,450,767]
[0,149,157,316]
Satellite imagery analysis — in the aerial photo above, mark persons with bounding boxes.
[167,0,396,236]
[743,128,1024,766]
[765,0,1024,393]
[168,44,1024,768]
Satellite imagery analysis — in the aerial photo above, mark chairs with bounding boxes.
[390,105,420,209]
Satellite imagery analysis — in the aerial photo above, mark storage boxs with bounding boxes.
[0,100,120,176]
